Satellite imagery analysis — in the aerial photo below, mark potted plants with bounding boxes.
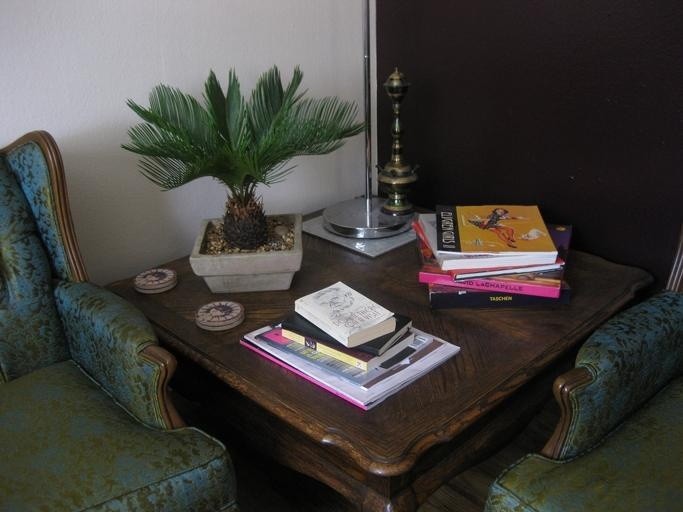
[123,62,365,295]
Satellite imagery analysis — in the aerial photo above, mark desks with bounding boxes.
[107,194,654,512]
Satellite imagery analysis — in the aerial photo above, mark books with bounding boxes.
[240,281,461,411]
[410,203,574,308]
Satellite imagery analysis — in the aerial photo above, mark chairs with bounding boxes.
[0,130,239,512]
[485,233,683,512]
